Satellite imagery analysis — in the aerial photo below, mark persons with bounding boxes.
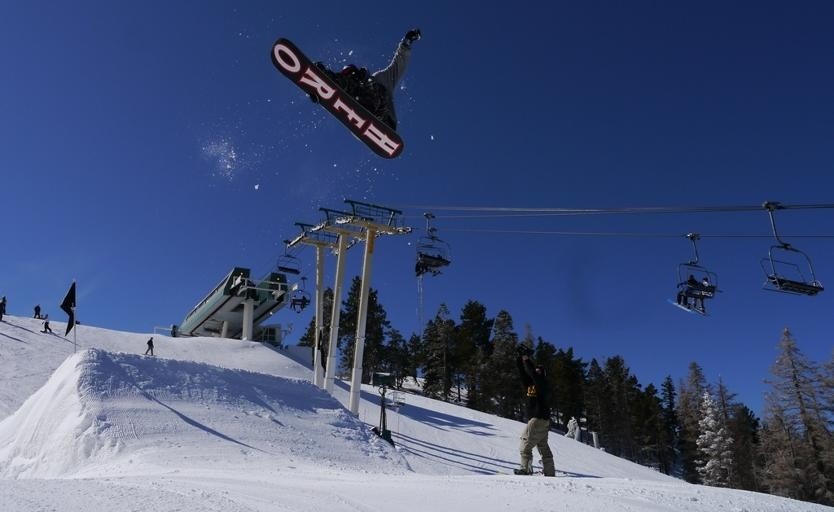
[300,296,306,310]
[692,277,711,312]
[145,336,155,356]
[33,304,41,319]
[40,314,52,333]
[290,295,297,311]
[0,296,6,321]
[514,354,556,477]
[676,275,698,304]
[315,26,422,132]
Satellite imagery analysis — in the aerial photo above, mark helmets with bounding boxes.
[535,363,547,377]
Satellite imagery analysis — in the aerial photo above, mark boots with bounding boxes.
[514,456,555,477]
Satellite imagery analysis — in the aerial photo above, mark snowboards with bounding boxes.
[668,300,694,314]
[689,304,711,316]
[270,37,403,159]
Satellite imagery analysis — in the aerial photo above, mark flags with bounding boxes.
[60,281,75,336]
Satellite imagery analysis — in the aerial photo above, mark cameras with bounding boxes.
[517,344,534,361]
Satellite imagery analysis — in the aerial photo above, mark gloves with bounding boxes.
[514,343,530,361]
